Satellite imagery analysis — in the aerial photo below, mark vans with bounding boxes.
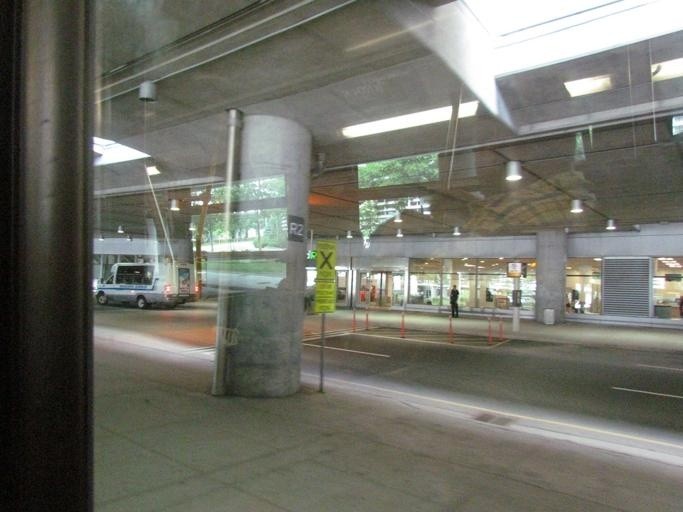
[95,261,198,310]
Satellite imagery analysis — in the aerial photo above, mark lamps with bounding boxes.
[96,199,182,242]
[331,150,617,241]
[138,79,158,103]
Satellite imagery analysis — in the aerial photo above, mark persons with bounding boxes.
[452,286,462,318]
[486,287,491,300]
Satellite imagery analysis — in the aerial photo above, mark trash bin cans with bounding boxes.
[544,308,555,325]
